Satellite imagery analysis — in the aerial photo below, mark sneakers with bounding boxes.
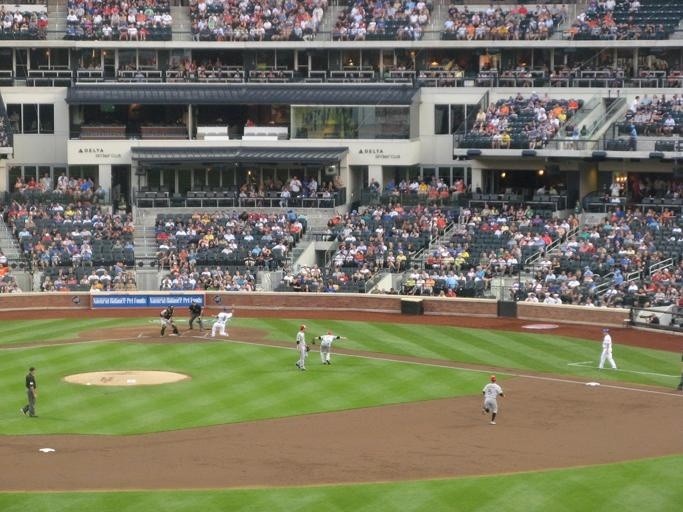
[326,359,330,365]
[296,363,300,369]
[491,421,496,425]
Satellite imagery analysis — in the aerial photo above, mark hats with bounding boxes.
[300,325,304,330]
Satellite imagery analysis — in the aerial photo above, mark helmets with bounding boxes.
[168,305,173,311]
[491,376,496,381]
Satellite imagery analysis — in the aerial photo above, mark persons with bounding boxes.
[596,326,616,370]
[20,367,39,417]
[1,1,682,87]
[208,302,234,337]
[313,328,348,365]
[0,170,683,326]
[293,324,311,371]
[157,303,180,337]
[464,92,682,152]
[479,374,506,425]
